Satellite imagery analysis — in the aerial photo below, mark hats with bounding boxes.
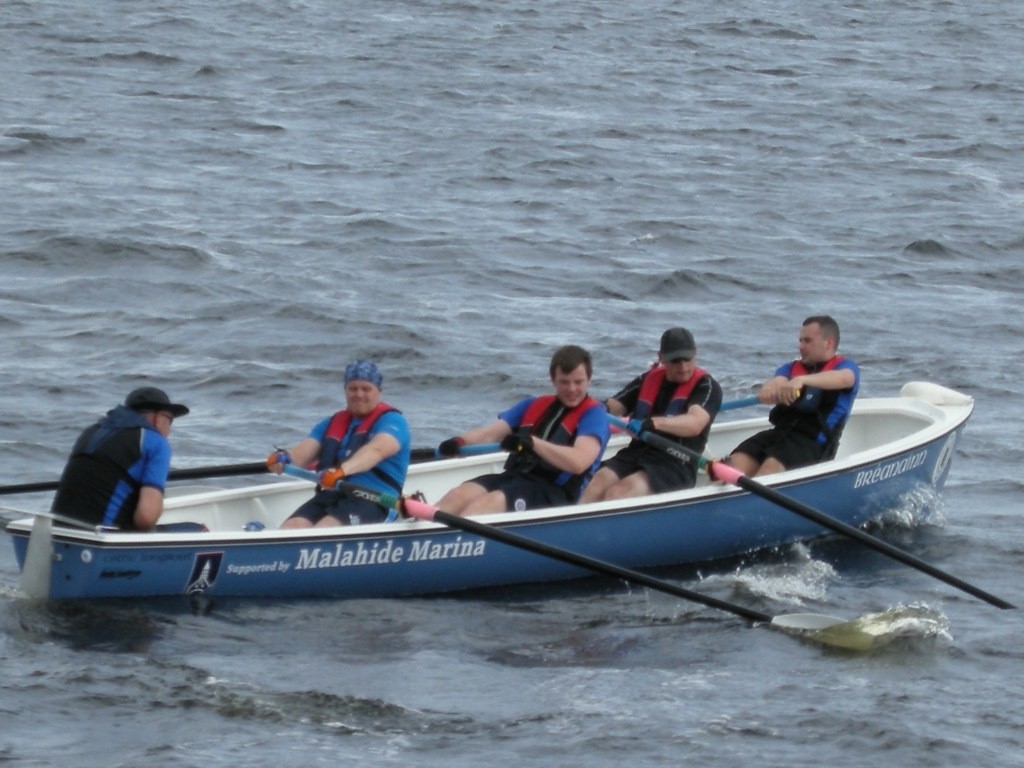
[661,327,696,362]
[125,387,191,418]
[344,359,383,387]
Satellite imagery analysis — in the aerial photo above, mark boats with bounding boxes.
[0,381,981,590]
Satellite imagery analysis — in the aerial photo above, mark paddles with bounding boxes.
[277,456,950,655]
[0,456,284,501]
[601,408,1019,611]
[718,388,802,412]
[407,440,512,465]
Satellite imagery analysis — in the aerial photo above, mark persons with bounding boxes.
[435,346,610,518]
[268,359,411,530]
[723,315,860,486]
[576,326,722,503]
[50,386,208,533]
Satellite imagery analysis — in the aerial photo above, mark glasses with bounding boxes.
[670,358,691,364]
[159,413,173,423]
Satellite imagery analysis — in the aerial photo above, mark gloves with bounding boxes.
[625,418,654,440]
[266,448,293,475]
[317,465,345,491]
[439,437,465,457]
[500,432,534,454]
